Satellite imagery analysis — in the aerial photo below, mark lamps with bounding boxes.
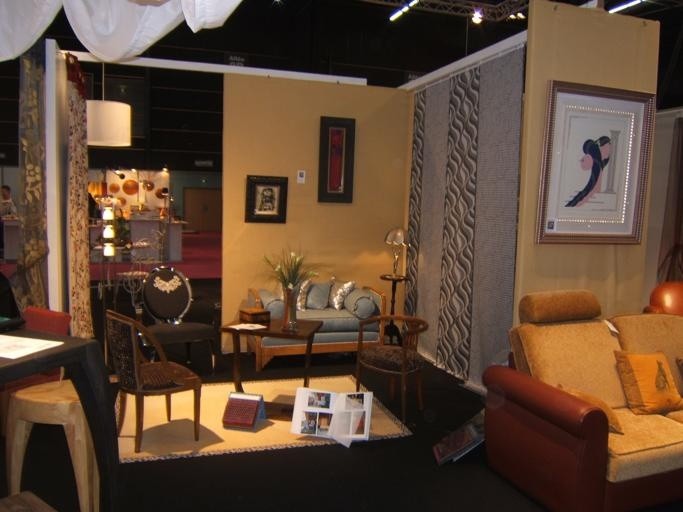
[379,228,408,346]
[83,58,132,147]
[102,206,115,256]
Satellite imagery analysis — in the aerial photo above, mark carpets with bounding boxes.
[117,374,412,466]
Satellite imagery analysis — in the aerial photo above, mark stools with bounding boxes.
[5,377,100,511]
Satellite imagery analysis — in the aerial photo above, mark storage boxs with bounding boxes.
[239,309,270,323]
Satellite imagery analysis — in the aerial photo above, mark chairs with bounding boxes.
[143,266,215,372]
[106,310,202,453]
[2,306,71,414]
[352,315,429,425]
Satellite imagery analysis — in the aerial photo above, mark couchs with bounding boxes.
[482,279,682,508]
[245,281,388,370]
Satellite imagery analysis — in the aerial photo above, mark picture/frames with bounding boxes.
[317,116,356,204]
[246,175,288,224]
[182,188,221,231]
[535,79,655,245]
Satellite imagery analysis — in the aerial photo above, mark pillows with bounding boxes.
[305,282,331,308]
[329,276,355,311]
[559,381,625,433]
[345,287,376,320]
[287,281,313,312]
[612,349,683,414]
[259,286,286,319]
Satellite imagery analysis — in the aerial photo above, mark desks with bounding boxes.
[220,317,323,393]
[93,240,157,308]
[1,327,117,460]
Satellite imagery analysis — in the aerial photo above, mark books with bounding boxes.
[435,407,486,466]
[290,387,373,448]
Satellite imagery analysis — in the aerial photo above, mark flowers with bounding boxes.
[262,251,320,319]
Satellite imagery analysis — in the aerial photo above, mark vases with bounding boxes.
[282,286,300,336]
